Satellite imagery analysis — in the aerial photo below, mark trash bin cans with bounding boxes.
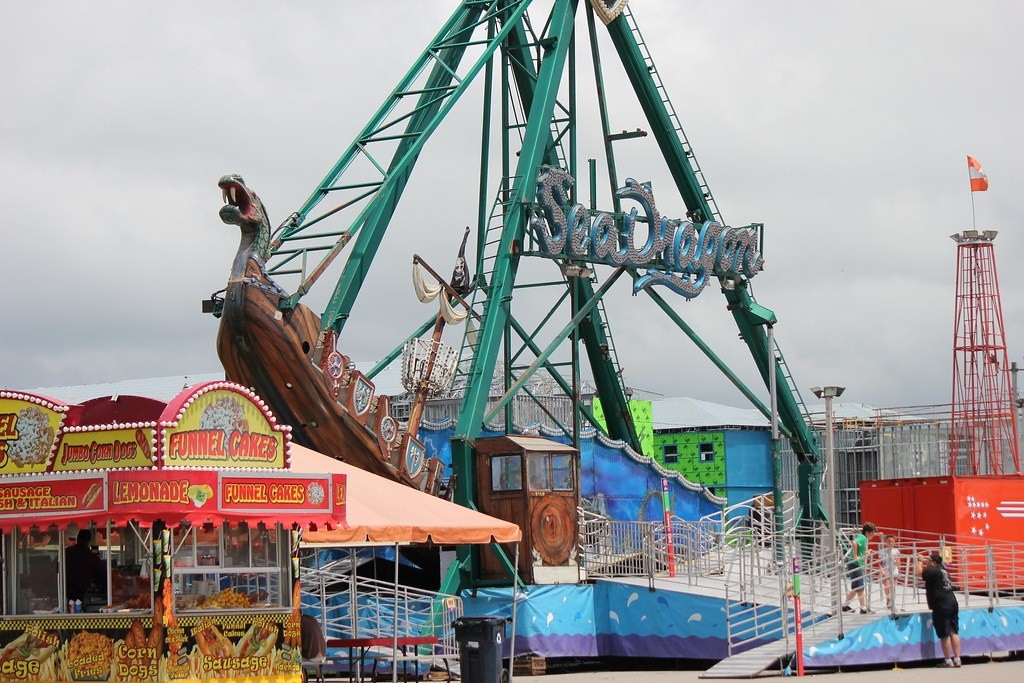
[451,615,513,683]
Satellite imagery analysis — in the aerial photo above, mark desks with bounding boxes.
[327,637,438,683]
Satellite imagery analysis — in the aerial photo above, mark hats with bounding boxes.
[930,554,943,564]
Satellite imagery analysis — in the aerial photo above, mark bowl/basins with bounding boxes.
[186,580,220,594]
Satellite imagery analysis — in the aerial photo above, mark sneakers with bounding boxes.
[935,659,954,668]
[860,608,875,614]
[842,605,855,613]
[954,658,962,667]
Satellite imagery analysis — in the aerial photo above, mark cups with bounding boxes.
[153,539,161,565]
[153,568,162,592]
[163,555,171,577]
[162,530,170,552]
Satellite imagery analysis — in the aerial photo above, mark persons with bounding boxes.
[301,609,325,661]
[917,555,961,667]
[842,522,877,613]
[41,527,107,603]
[878,535,900,608]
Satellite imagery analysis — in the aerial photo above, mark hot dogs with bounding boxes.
[194,622,279,663]
[0,627,59,678]
[82,484,102,509]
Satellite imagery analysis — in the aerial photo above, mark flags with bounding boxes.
[968,156,988,191]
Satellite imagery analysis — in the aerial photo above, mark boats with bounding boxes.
[195,172,822,587]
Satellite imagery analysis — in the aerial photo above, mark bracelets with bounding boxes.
[918,559,922,561]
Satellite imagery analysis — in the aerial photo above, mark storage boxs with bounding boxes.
[859,473,1023,594]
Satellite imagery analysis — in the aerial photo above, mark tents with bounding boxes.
[286,440,523,683]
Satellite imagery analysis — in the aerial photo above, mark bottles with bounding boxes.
[197,547,216,565]
[68,599,75,613]
[75,599,82,613]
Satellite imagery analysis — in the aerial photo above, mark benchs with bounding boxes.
[302,655,458,683]
